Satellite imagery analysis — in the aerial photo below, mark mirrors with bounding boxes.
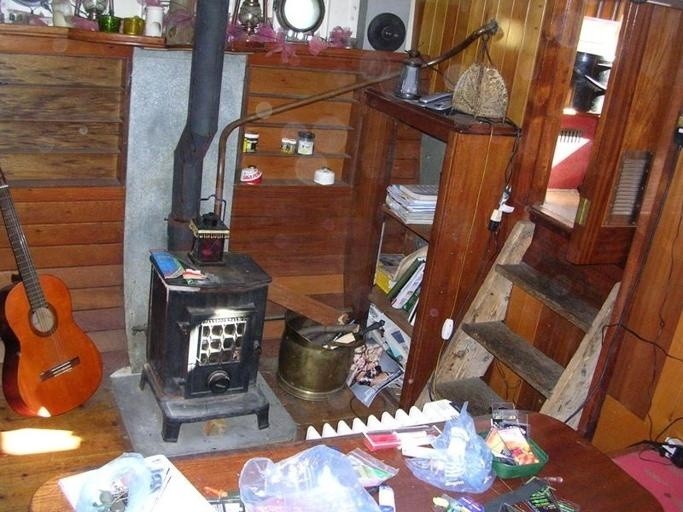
[277,0,325,44]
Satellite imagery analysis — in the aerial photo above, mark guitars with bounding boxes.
[0,168,102,418]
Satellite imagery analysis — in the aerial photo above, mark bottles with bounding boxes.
[394,49,422,101]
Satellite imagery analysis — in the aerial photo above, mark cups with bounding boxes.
[96,13,122,34]
[118,16,145,37]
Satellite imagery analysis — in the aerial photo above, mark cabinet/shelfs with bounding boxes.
[233,63,363,188]
[1,48,133,187]
[343,88,523,415]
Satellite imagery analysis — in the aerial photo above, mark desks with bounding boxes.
[31,409,664,512]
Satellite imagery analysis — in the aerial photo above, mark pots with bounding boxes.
[274,309,366,404]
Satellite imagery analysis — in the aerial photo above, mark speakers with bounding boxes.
[356,0,416,53]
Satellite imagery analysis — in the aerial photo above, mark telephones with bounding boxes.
[415,92,453,111]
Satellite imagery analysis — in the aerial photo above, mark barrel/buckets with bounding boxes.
[276,309,355,403]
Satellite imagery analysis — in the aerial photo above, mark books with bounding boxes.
[385,182,440,225]
